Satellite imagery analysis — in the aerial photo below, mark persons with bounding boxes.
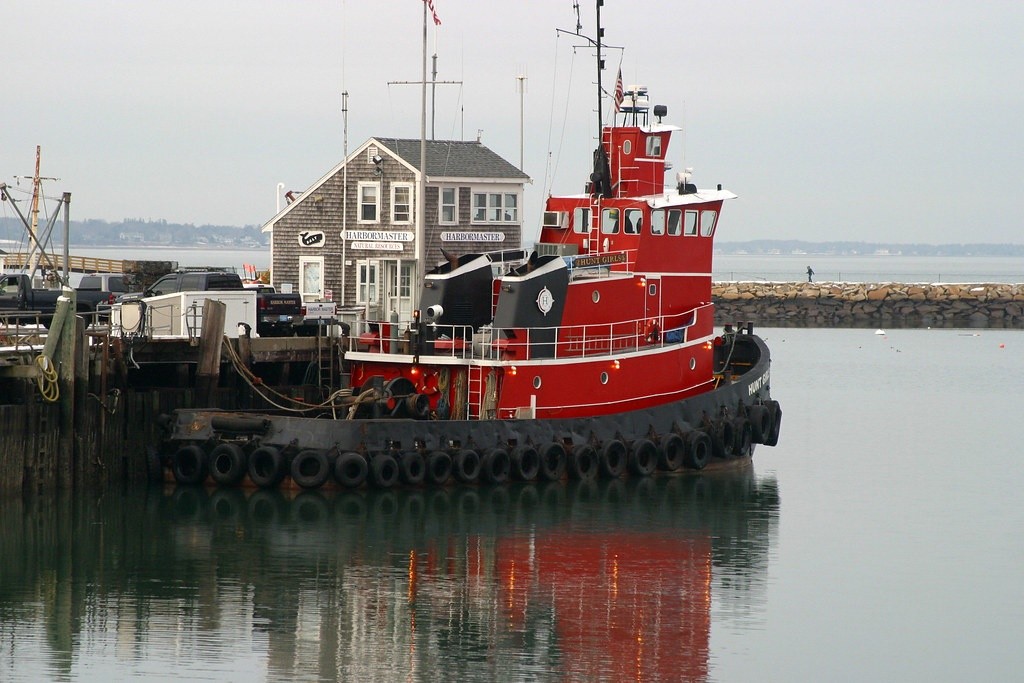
[804,266,815,282]
[614,210,655,234]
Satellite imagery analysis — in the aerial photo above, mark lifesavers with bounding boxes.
[484,447,514,485]
[370,456,399,488]
[456,449,481,480]
[644,318,662,343]
[427,451,455,484]
[401,451,426,485]
[511,400,783,484]
[335,453,369,488]
[291,448,331,489]
[247,446,287,486]
[171,444,206,485]
[206,441,247,486]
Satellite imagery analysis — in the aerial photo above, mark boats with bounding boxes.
[153,0,783,491]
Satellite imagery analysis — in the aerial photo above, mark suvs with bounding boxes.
[79,273,128,318]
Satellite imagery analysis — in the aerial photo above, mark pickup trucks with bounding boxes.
[113,266,307,336]
[241,282,337,338]
[0,272,115,332]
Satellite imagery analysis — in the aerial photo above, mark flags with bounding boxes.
[427,0,441,26]
[614,69,625,114]
